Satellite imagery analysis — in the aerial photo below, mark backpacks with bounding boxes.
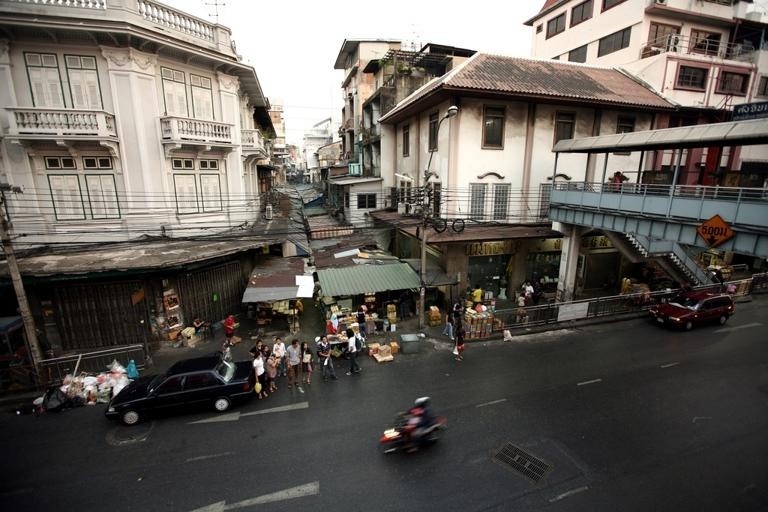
[354,336,362,351]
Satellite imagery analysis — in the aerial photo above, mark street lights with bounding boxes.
[419,106,458,329]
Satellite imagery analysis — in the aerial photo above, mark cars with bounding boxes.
[646,288,736,330]
[104,350,256,427]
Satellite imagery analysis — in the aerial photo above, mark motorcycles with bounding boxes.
[378,412,448,456]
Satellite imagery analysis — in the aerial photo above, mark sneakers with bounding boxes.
[258,367,363,400]
[454,355,463,362]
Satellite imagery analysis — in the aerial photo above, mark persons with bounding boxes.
[471,284,482,309]
[356,302,367,339]
[442,303,466,361]
[612,171,623,192]
[396,393,436,454]
[608,172,629,193]
[248,334,338,396]
[224,315,237,347]
[345,329,360,375]
[256,311,266,336]
[516,278,541,327]
[614,263,736,311]
[194,316,205,335]
[296,298,304,330]
[334,305,342,319]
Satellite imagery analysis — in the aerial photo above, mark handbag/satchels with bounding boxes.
[254,381,262,394]
[452,346,459,354]
